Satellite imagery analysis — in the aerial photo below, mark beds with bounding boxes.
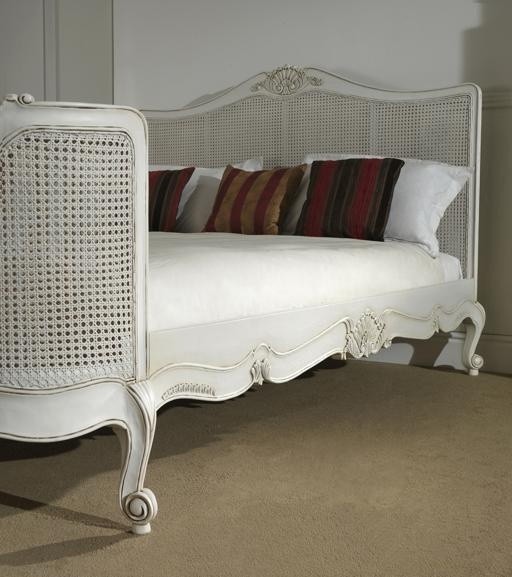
[1,64,485,534]
